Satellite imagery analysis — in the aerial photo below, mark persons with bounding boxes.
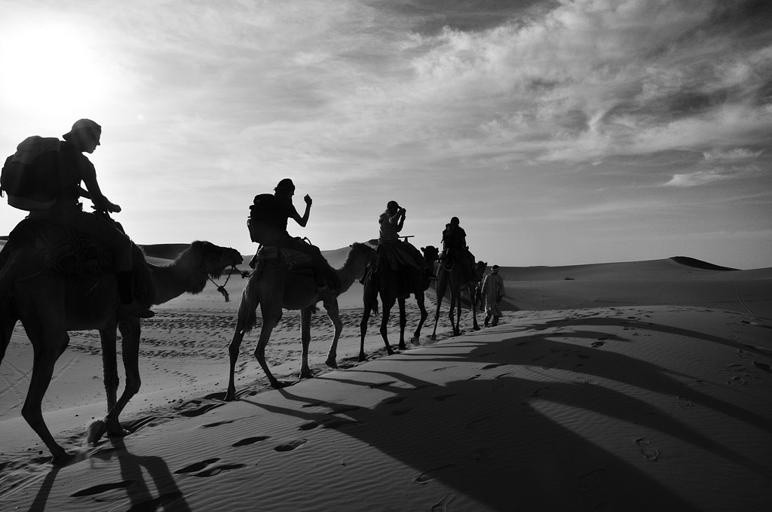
[481,265,504,326]
[378,200,426,277]
[32,119,154,318]
[249,179,336,295]
[443,217,467,251]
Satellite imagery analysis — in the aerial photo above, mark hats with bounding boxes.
[62,119,101,141]
[387,201,402,210]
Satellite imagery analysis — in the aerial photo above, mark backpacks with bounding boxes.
[0,136,62,210]
[247,194,281,242]
